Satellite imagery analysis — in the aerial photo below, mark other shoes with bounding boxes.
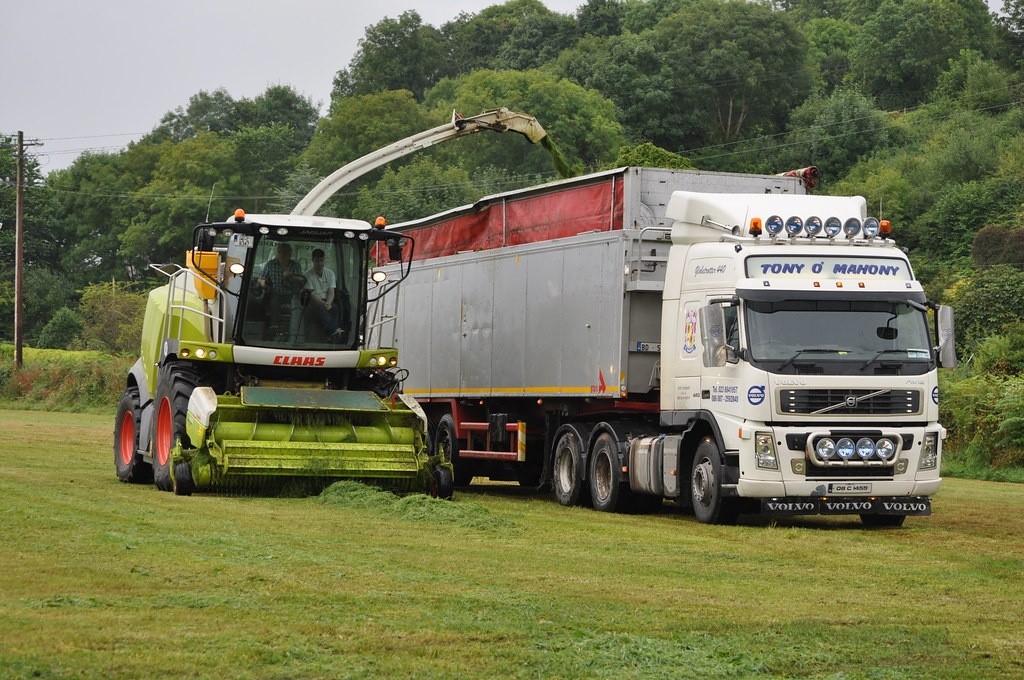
[328,328,345,343]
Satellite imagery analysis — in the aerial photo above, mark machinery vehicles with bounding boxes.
[112,107,548,496]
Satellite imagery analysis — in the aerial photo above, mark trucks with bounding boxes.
[359,166,947,531]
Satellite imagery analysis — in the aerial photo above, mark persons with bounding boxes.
[301,248,346,343]
[257,243,303,341]
